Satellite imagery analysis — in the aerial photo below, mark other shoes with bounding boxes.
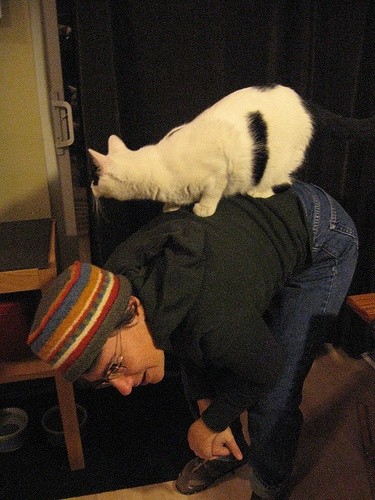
[175,441,251,494]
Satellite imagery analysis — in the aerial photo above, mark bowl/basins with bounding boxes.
[41,403,92,457]
[0,407,29,453]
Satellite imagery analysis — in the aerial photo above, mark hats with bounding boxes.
[26,261,131,384]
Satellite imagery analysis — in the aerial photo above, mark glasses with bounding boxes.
[93,327,126,390]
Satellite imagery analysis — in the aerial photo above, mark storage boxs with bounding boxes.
[0,293,35,360]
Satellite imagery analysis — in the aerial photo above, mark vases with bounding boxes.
[42,404,87,439]
[0,407,29,454]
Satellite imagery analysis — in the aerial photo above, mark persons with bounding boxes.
[24,176,361,500]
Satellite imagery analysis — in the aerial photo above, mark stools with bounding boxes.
[339,292,375,366]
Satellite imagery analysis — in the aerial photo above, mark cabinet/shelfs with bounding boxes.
[0,218,85,471]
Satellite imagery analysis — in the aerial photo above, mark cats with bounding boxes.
[85,83,315,219]
